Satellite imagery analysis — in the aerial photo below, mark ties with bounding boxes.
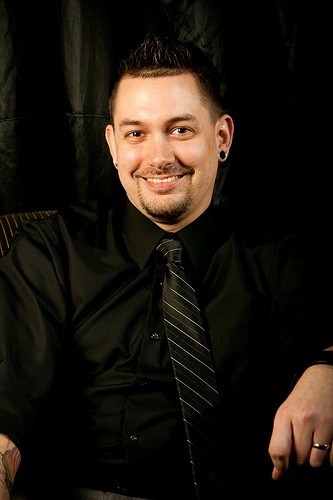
[155,238,235,500]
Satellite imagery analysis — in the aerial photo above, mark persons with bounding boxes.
[0,36,333,500]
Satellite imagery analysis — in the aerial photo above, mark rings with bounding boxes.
[312,442,329,450]
